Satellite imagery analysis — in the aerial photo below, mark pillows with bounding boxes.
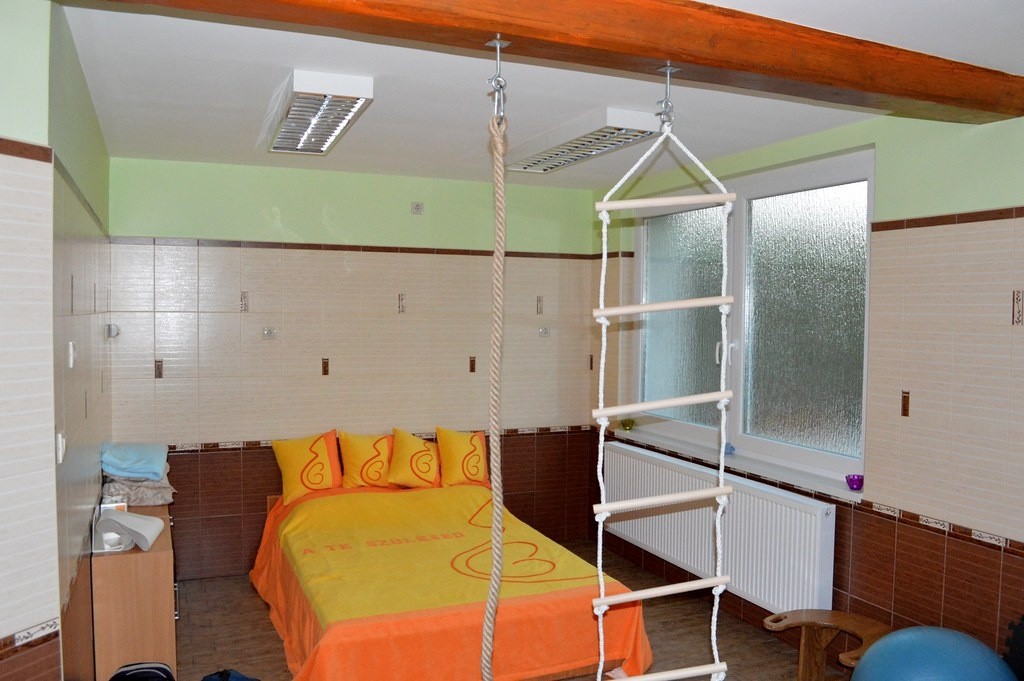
[338,430,399,489]
[436,426,491,489]
[387,427,442,488]
[273,428,344,506]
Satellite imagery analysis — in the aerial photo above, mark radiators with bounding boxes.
[603,442,837,615]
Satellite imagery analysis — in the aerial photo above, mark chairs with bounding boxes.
[848,627,1019,680]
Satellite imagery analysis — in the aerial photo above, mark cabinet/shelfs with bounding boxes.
[92,504,177,681]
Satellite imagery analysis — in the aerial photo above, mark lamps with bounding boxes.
[503,107,667,174]
[268,69,373,156]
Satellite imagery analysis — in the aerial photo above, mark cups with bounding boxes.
[621,419,634,430]
[846,474,863,490]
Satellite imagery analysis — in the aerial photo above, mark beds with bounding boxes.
[248,482,654,681]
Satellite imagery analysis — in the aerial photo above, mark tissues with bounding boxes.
[100,493,129,516]
